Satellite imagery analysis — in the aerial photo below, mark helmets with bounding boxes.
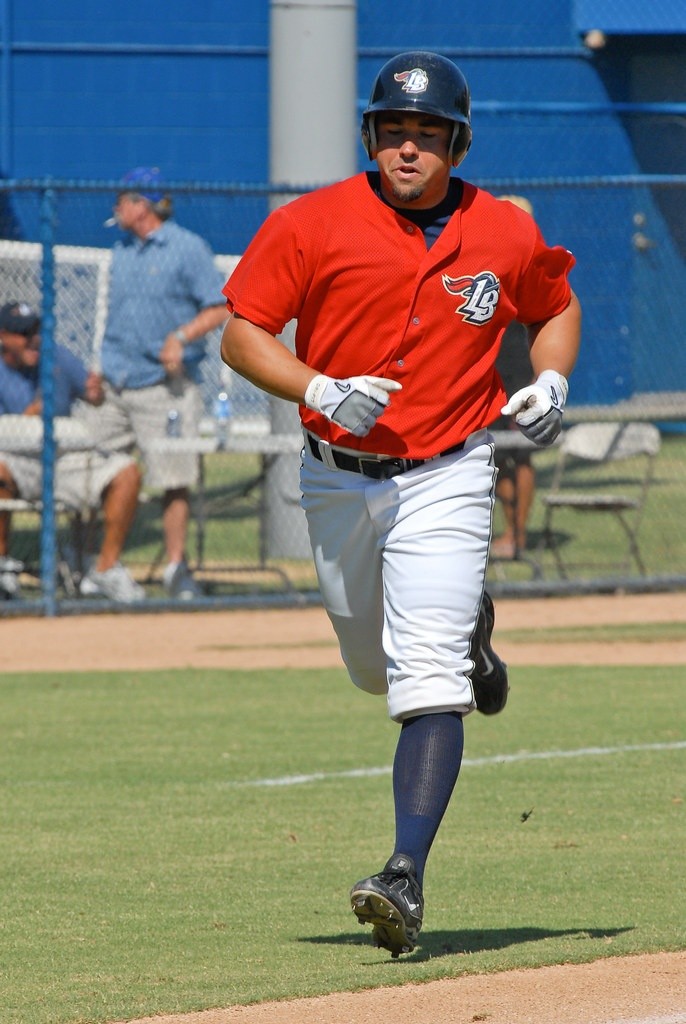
[362,51,472,126]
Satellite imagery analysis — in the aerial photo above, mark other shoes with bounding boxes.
[164,561,202,602]
[0,559,24,595]
[78,562,145,603]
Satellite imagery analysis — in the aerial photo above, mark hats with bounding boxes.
[0,299,40,331]
[116,167,173,204]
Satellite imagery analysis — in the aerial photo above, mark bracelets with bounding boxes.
[173,330,186,348]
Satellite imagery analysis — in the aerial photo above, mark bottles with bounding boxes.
[213,390,231,440]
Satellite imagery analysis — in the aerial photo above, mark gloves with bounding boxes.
[305,373,403,438]
[500,370,568,446]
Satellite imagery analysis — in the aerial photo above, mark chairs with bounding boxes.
[0,499,83,586]
[533,421,661,580]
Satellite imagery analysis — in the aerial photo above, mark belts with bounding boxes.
[308,432,465,480]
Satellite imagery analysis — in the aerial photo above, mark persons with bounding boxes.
[487,196,535,561]
[220,50,582,958]
[0,166,231,606]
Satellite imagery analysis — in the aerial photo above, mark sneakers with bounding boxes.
[469,591,509,714]
[349,853,424,958]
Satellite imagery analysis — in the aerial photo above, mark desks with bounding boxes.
[146,429,564,596]
